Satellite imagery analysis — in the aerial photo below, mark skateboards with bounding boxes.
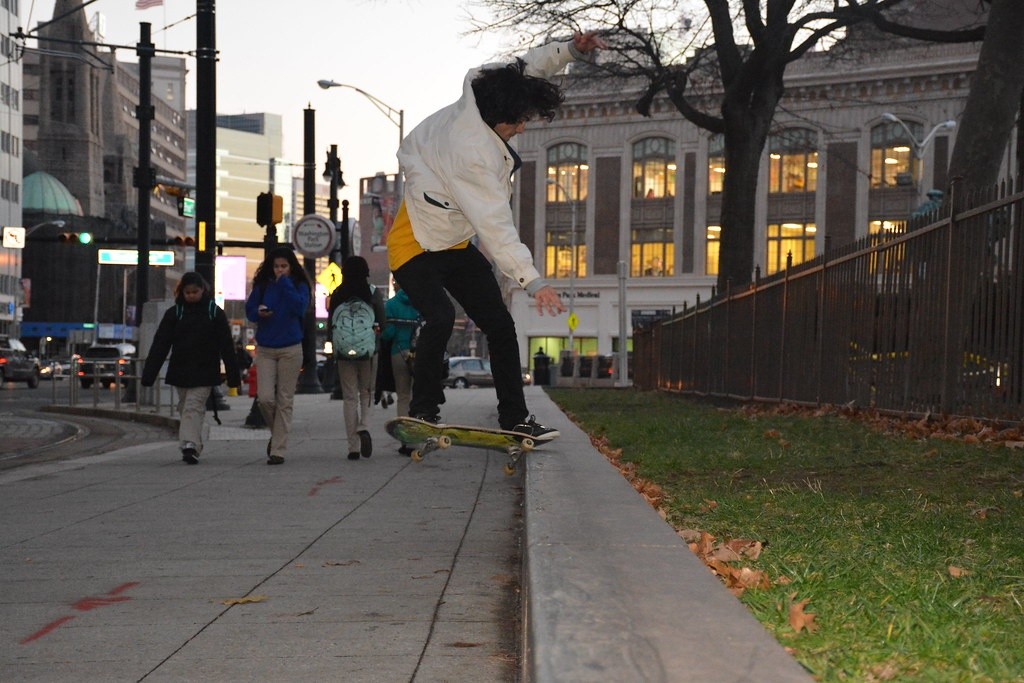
[382,415,557,477]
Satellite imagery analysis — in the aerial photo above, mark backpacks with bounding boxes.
[330,283,377,360]
[391,314,450,381]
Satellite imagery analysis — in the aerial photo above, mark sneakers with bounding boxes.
[514,415,561,440]
[411,412,442,425]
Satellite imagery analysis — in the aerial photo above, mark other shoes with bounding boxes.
[266,436,272,455]
[182,442,199,465]
[397,446,415,456]
[348,452,361,460]
[266,456,284,465]
[381,395,394,409]
[358,430,372,459]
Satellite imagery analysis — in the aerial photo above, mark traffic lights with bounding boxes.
[174,236,195,246]
[257,192,282,226]
[56,233,94,244]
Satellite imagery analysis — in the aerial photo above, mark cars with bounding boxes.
[445,356,532,389]
[80,343,136,389]
[0,333,41,389]
[38,355,81,381]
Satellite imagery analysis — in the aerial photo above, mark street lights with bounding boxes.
[881,112,956,208]
[12,220,66,339]
[546,179,574,357]
[323,145,345,263]
[318,79,404,203]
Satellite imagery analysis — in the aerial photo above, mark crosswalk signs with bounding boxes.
[568,313,578,331]
[316,262,343,295]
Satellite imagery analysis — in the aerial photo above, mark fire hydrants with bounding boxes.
[244,363,258,398]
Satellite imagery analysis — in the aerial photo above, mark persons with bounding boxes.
[245,246,313,464]
[327,255,385,461]
[235,336,253,370]
[381,282,425,455]
[535,347,545,356]
[139,272,241,464]
[385,31,610,445]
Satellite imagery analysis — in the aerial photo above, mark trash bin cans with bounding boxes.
[533,354,552,386]
